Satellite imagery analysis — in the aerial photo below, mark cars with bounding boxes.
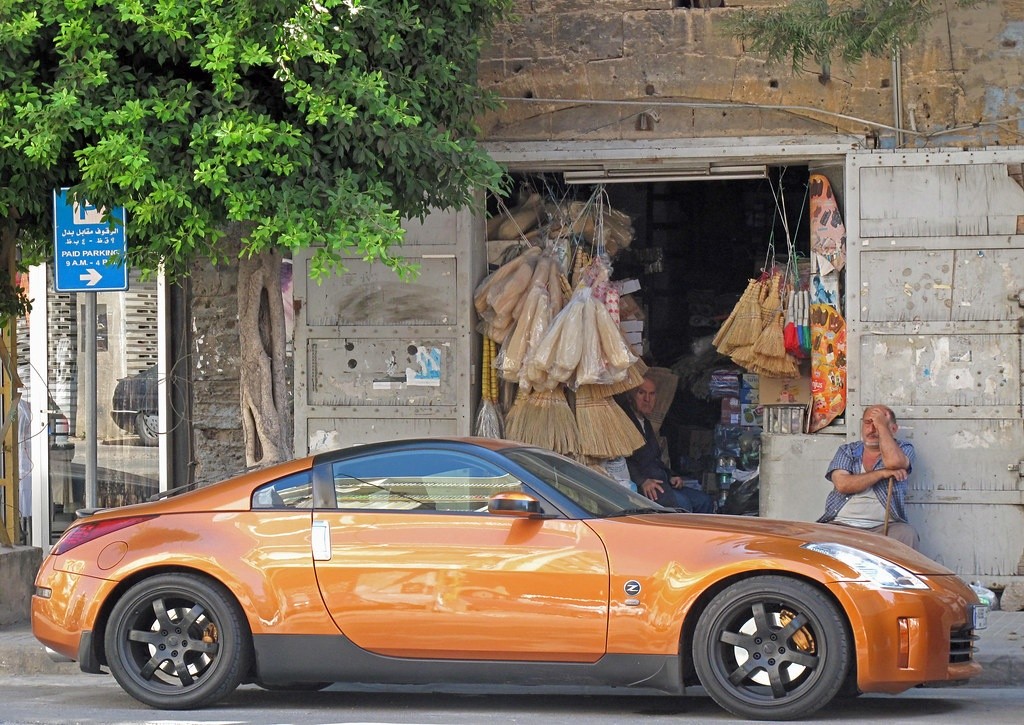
[109,351,159,448]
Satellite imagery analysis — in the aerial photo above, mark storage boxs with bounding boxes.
[758,359,810,406]
[678,424,713,457]
[720,373,763,426]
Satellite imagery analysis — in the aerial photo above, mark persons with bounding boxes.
[815,404,920,552]
[812,276,836,309]
[619,377,713,514]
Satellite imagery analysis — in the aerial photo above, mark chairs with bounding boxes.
[388,476,436,511]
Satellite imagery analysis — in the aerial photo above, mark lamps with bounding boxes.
[563,165,769,185]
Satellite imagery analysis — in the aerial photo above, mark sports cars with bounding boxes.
[27,433,985,722]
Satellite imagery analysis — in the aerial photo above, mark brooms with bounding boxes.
[711,272,802,379]
[473,247,646,465]
